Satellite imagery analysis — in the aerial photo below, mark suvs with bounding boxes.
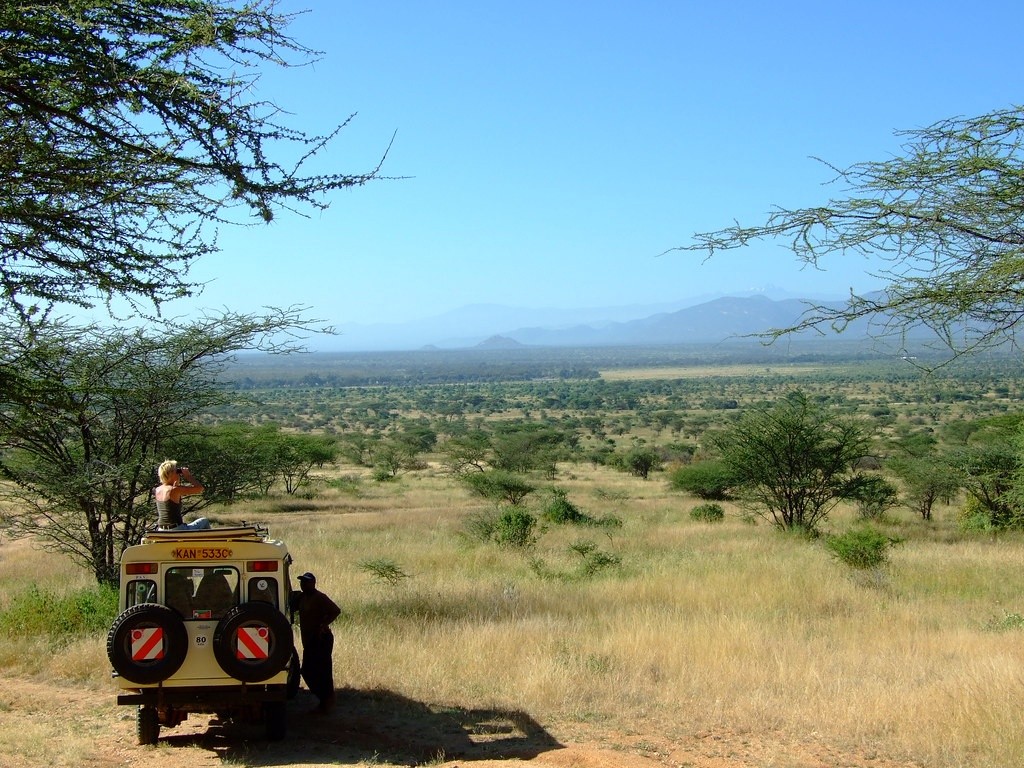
[106,520,302,744]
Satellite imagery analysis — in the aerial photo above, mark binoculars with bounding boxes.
[176,469,191,474]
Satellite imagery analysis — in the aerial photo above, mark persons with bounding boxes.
[292,573,341,714]
[155,460,212,531]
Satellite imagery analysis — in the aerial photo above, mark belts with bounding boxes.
[159,522,182,529]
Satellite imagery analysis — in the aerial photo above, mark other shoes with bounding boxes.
[310,693,336,713]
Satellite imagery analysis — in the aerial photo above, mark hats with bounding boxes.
[297,573,316,582]
